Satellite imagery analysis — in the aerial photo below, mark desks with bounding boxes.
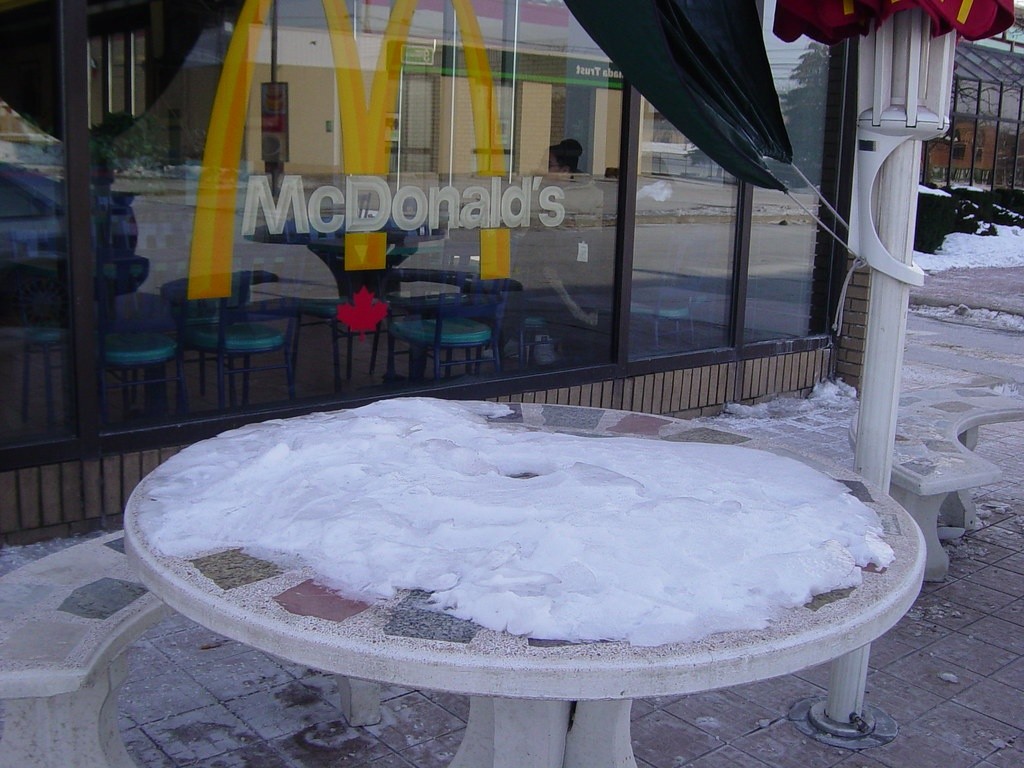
[307,237,501,385]
[57,248,284,419]
[122,396,927,768]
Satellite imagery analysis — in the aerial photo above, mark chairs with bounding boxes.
[630,229,718,353]
[96,249,185,424]
[106,202,185,251]
[184,244,311,411]
[12,227,70,425]
[283,220,397,389]
[389,237,519,383]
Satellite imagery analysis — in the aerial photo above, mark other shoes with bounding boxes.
[484,338,520,359]
[534,334,554,365]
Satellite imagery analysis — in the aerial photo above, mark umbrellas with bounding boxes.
[773,1,1017,724]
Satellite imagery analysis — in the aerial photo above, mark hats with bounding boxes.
[550,139,583,169]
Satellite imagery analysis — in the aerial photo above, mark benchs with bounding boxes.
[0,530,381,768]
[849,397,1024,582]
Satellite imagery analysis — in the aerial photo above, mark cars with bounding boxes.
[0,162,149,324]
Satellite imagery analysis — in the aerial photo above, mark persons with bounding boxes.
[503,141,615,365]
[51,111,149,439]
